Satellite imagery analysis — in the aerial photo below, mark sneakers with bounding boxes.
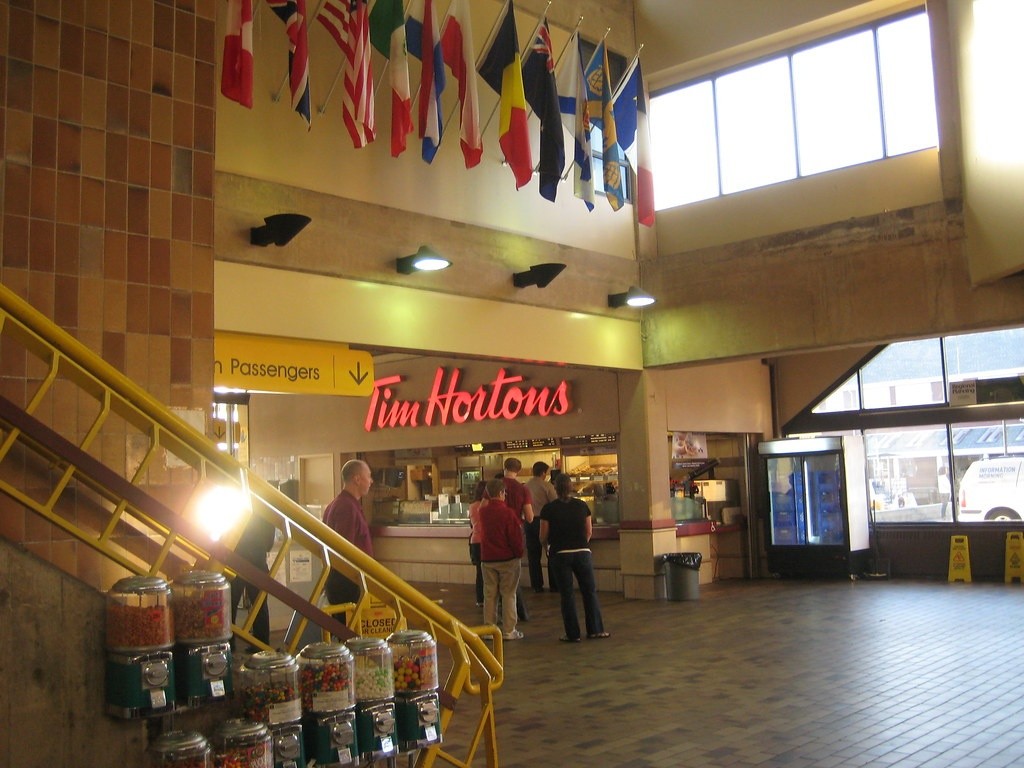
[586,631,610,638]
[502,628,525,640]
[559,632,581,642]
[481,634,493,639]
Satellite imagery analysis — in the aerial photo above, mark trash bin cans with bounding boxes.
[662,552,703,600]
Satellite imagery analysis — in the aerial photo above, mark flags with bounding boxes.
[475,0,533,191]
[584,37,624,212]
[554,31,594,213]
[368,0,414,158]
[521,18,565,202]
[317,0,376,148]
[440,0,483,169]
[405,0,446,165]
[221,0,254,109]
[612,55,655,227]
[267,0,312,131]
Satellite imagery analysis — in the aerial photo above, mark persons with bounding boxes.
[539,474,610,642]
[938,467,950,519]
[525,461,559,594]
[322,460,373,626]
[470,457,534,640]
[221,512,275,653]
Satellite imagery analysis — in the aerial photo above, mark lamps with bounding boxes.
[253,212,313,248]
[609,286,654,309]
[512,261,568,292]
[396,244,453,275]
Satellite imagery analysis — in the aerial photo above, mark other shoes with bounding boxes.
[476,601,484,607]
[244,647,253,653]
[533,587,547,593]
